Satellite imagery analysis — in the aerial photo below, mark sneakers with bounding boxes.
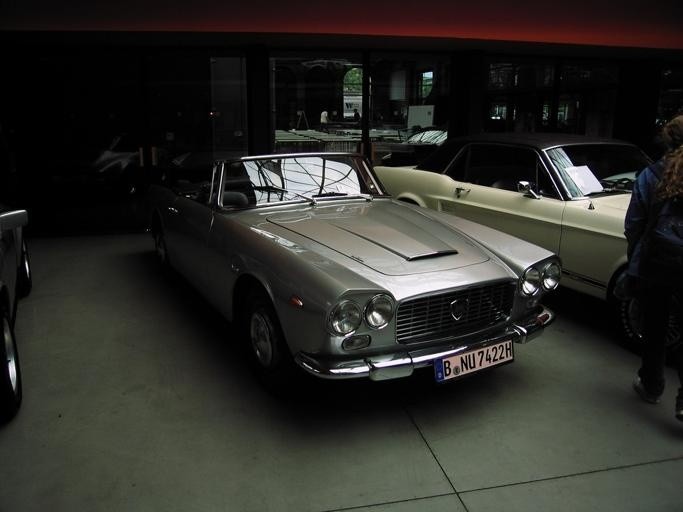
[674,406,683,421]
[632,377,661,404]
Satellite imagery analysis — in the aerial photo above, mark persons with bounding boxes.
[624,113,683,421]
[320,107,330,134]
[353,108,360,128]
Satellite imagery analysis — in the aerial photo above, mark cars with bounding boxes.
[144,150,564,383]
[0,198,34,424]
[369,133,649,354]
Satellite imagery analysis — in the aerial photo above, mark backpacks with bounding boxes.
[649,162,682,270]
